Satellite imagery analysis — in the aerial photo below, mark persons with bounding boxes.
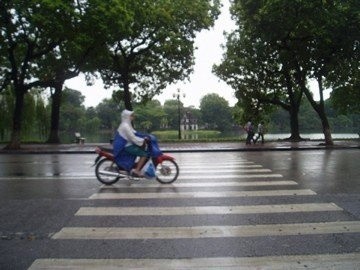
[255,120,264,144]
[113,110,149,177]
[244,120,255,145]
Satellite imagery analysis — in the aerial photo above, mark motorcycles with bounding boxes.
[94,137,179,185]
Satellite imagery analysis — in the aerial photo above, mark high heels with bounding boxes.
[130,168,145,177]
